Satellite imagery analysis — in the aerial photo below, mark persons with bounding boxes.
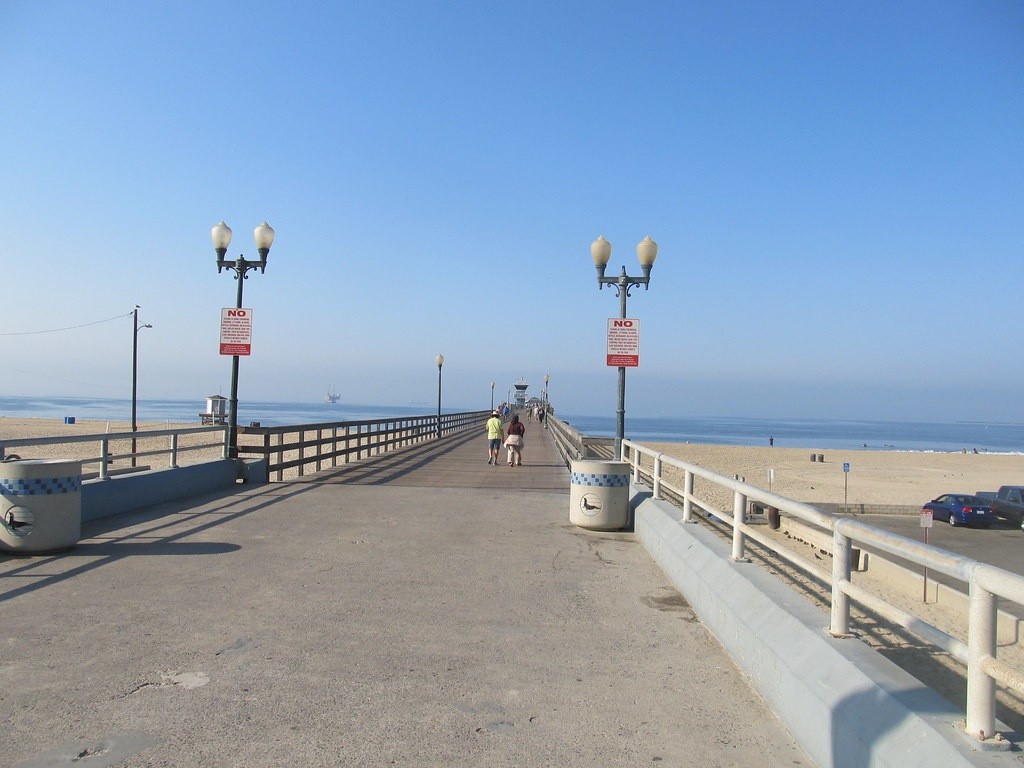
[532,402,545,423]
[486,410,505,466]
[503,415,526,467]
[769,435,774,447]
[496,401,509,420]
[525,405,532,424]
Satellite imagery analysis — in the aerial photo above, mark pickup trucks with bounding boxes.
[975,485,1024,530]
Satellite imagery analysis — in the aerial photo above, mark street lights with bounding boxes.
[544,374,550,429]
[435,353,445,440]
[490,382,495,415]
[130,323,153,467]
[210,220,277,460]
[590,234,658,460]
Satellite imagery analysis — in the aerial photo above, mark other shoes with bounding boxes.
[517,463,521,465]
[510,463,513,467]
[488,458,492,465]
[493,462,498,465]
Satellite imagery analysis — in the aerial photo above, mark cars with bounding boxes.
[923,493,996,528]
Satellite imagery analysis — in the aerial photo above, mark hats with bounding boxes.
[492,411,498,415]
